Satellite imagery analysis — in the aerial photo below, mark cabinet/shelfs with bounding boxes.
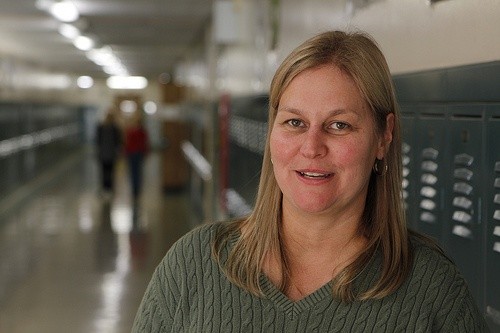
[224,60,500,333]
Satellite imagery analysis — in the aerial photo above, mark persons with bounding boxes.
[93,112,149,194]
[132,30,490,333]
